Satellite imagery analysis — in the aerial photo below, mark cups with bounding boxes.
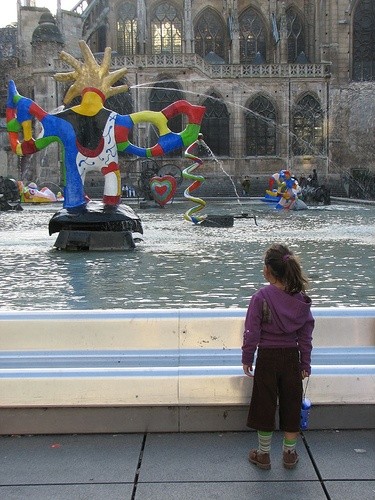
[301,396,311,429]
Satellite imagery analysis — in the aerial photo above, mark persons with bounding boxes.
[299,173,307,188]
[311,169,318,183]
[0,176,4,183]
[306,175,312,182]
[240,242,315,471]
[241,175,251,197]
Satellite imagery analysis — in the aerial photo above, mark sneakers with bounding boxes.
[283,450,299,468]
[248,449,271,469]
